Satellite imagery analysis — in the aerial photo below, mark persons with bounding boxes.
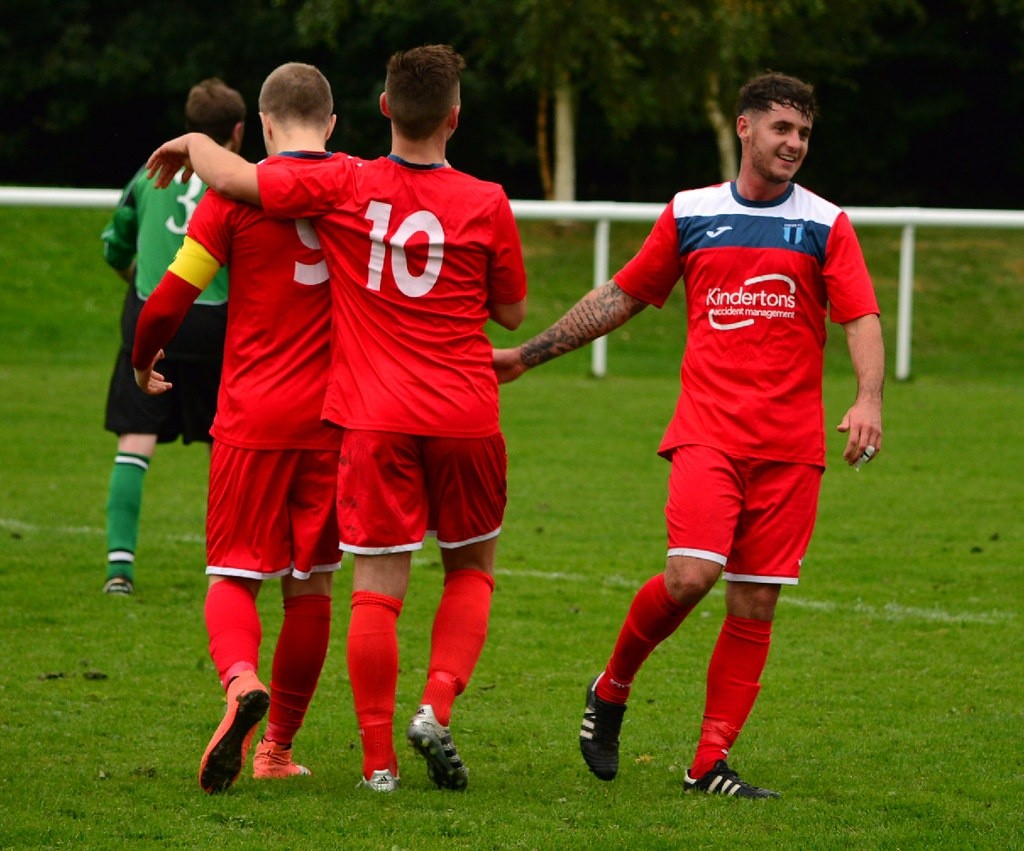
[132,62,354,792]
[145,44,526,792]
[492,75,885,800]
[99,78,245,597]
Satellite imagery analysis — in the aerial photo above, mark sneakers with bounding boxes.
[681,759,780,800]
[99,576,135,597]
[360,768,401,793]
[252,736,313,781]
[405,704,468,790]
[197,672,271,794]
[579,669,628,782]
